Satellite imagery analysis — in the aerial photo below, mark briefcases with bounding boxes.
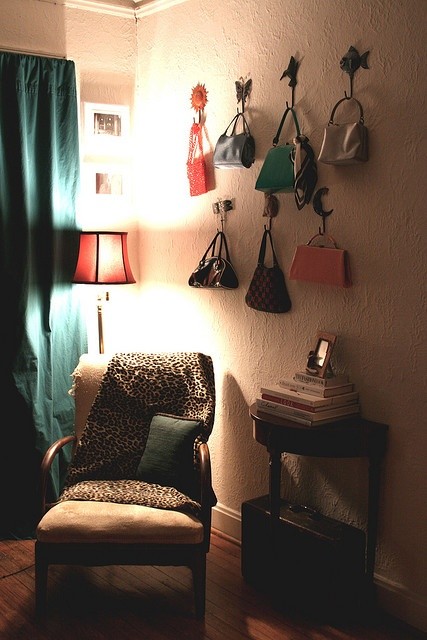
[240,494,365,618]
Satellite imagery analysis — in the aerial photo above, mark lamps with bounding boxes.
[70,231,136,353]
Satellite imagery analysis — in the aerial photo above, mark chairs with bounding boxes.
[34,354,209,622]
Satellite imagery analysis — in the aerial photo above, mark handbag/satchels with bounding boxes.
[255,107,318,211]
[317,96,370,167]
[212,113,255,170]
[188,231,238,290]
[186,123,209,196]
[288,233,352,288]
[245,229,292,313]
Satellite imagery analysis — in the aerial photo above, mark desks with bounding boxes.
[249,402,389,628]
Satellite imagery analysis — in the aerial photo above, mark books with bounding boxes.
[255,368,361,428]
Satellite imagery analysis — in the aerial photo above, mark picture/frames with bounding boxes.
[82,101,131,147]
[82,160,132,205]
[304,331,337,379]
